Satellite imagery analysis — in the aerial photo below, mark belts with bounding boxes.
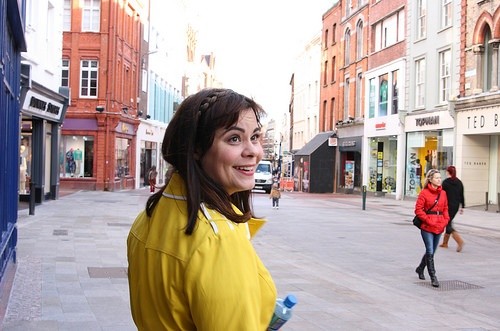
[426,211,443,215]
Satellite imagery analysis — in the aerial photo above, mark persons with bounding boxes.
[439,166,466,252]
[18,146,28,195]
[127,89,281,331]
[66,148,76,176]
[414,170,450,287]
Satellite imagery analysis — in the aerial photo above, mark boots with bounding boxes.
[416,253,428,280]
[439,233,451,248]
[451,230,464,252]
[428,253,439,287]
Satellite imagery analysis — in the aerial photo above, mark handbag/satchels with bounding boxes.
[413,215,423,229]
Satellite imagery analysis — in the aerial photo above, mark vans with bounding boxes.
[253,161,273,193]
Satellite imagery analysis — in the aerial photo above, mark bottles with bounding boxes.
[267,294,298,331]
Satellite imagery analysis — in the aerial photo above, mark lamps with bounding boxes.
[96,108,150,120]
